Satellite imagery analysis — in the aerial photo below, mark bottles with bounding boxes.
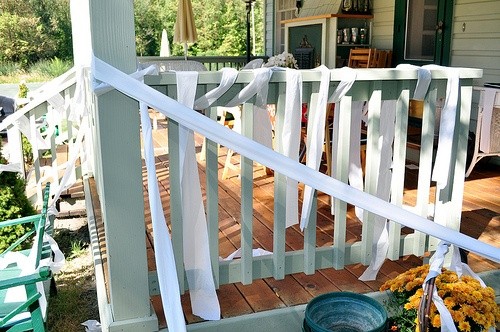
[363,20,369,44]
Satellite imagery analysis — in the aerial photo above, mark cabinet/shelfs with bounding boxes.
[328,13,373,67]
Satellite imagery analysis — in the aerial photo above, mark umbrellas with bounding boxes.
[173,0,199,60]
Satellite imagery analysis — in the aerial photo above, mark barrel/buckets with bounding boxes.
[303,291,388,332]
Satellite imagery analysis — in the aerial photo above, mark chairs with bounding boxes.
[348,47,391,70]
[296,100,367,211]
[200,59,263,181]
[0,181,57,332]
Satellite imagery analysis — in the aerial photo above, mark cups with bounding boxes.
[337,27,367,45]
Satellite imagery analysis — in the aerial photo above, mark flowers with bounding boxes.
[379,264,500,332]
[268,51,299,69]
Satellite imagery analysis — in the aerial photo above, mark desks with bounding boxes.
[465,86,500,178]
